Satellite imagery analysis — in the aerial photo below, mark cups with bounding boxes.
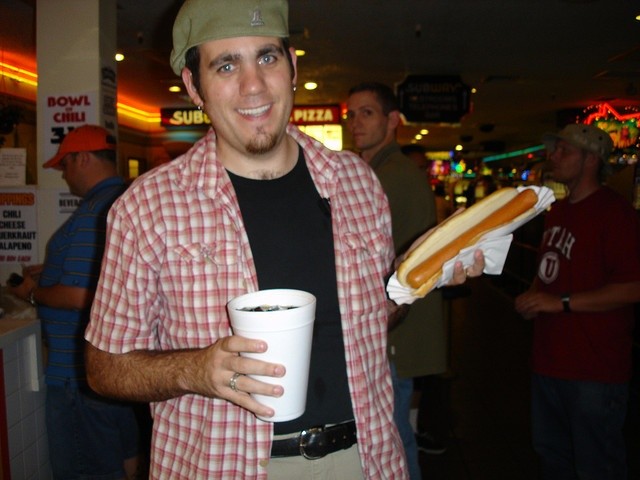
[226,288,316,423]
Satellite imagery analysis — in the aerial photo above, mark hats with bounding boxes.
[42,125,118,170]
[169,0,290,77]
[539,124,614,166]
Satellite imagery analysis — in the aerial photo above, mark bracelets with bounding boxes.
[28,286,39,305]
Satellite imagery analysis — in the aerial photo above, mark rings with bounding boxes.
[228,373,239,388]
[512,123,640,479]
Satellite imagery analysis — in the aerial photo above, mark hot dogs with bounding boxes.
[399,187,538,298]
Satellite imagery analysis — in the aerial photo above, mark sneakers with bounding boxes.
[415,433,446,454]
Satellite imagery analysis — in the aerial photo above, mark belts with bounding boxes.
[270,420,357,462]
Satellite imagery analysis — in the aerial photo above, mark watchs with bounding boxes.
[562,296,570,302]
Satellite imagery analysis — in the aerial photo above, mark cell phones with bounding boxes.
[6,273,23,286]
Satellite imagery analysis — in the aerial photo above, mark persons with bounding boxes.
[347,80,451,479]
[84,0,485,480]
[14,123,142,479]
